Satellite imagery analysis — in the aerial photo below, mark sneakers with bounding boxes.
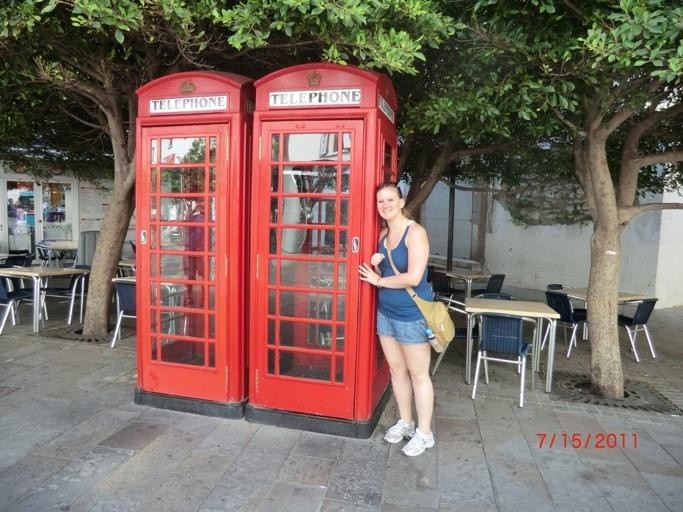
[382,419,416,445]
[400,427,435,457]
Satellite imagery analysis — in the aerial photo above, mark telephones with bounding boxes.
[271,166,314,268]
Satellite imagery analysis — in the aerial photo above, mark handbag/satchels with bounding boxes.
[424,300,456,354]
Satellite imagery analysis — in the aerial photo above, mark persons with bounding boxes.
[357,181,434,457]
[181,192,213,307]
[8,198,15,216]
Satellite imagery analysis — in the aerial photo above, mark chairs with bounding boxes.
[0,241,178,347]
[426,262,659,408]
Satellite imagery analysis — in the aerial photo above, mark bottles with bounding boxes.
[425,329,442,353]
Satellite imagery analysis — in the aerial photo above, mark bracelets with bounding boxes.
[376,277,381,288]
